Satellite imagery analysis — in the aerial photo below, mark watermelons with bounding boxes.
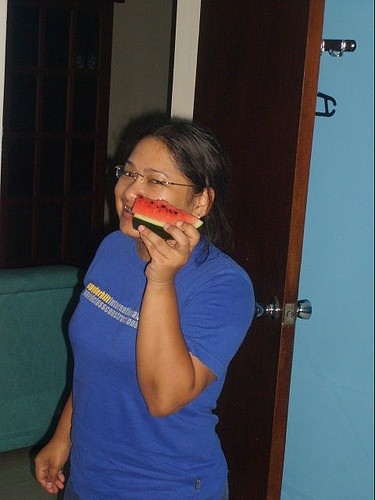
[131,195,205,240]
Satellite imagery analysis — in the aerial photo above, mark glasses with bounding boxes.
[115,165,196,188]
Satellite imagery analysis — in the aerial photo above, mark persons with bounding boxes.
[34,119,255,500]
